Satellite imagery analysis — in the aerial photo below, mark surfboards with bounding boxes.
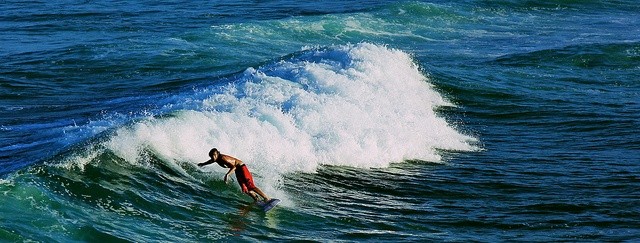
[254,199,279,212]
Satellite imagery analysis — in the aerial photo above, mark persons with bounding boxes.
[197,148,271,204]
